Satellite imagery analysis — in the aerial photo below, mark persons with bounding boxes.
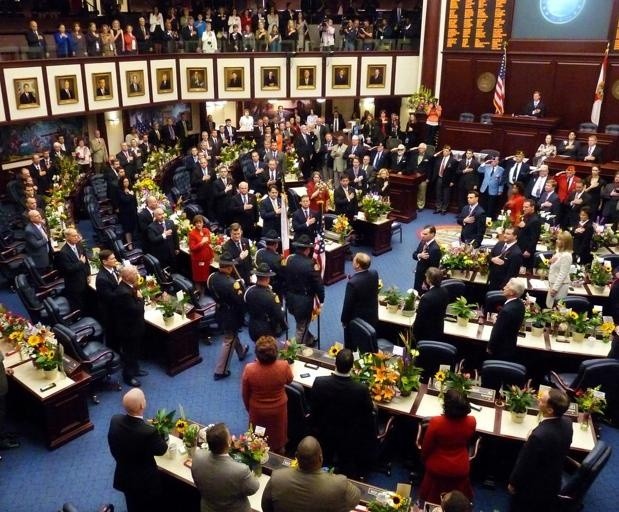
[20,83,37,104]
[159,73,170,90]
[413,224,442,296]
[104,105,420,380]
[456,131,619,310]
[419,389,475,503]
[411,266,447,345]
[228,72,241,87]
[503,389,574,512]
[370,68,383,84]
[108,387,169,512]
[190,422,261,512]
[23,5,411,53]
[424,99,442,144]
[190,71,202,88]
[264,71,277,86]
[60,79,75,99]
[241,335,294,457]
[17,128,150,389]
[486,276,526,364]
[97,78,110,96]
[261,436,361,512]
[306,348,377,479]
[335,70,346,85]
[130,75,141,92]
[406,142,457,215]
[526,90,546,118]
[300,69,313,85]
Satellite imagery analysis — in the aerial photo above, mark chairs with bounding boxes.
[1,113,618,512]
[0,0,419,55]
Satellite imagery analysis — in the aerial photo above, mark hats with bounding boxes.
[254,262,276,277]
[214,251,238,265]
[261,230,281,242]
[291,234,313,247]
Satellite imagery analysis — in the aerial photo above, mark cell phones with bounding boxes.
[300,373,310,378]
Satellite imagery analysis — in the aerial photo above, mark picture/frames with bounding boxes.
[117,59,153,108]
[147,58,179,105]
[2,65,51,123]
[324,56,358,98]
[177,57,216,102]
[253,57,288,100]
[214,57,252,101]
[359,56,393,98]
[44,63,89,116]
[289,56,324,99]
[82,62,122,113]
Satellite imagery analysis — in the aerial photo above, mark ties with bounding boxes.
[272,171,274,180]
[305,210,308,220]
[243,196,247,202]
[441,159,444,177]
[513,164,518,182]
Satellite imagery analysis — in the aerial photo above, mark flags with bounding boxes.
[590,51,607,127]
[493,55,506,115]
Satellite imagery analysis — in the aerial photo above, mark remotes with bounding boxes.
[470,402,481,411]
[41,383,56,391]
[305,362,319,370]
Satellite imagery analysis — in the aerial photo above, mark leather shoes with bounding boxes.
[239,344,249,360]
[124,369,148,387]
[215,370,231,380]
